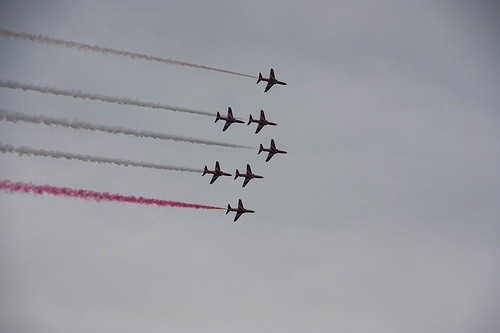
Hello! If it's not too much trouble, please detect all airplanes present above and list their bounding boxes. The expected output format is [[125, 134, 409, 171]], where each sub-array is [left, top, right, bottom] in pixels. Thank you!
[[201, 68, 288, 223]]
